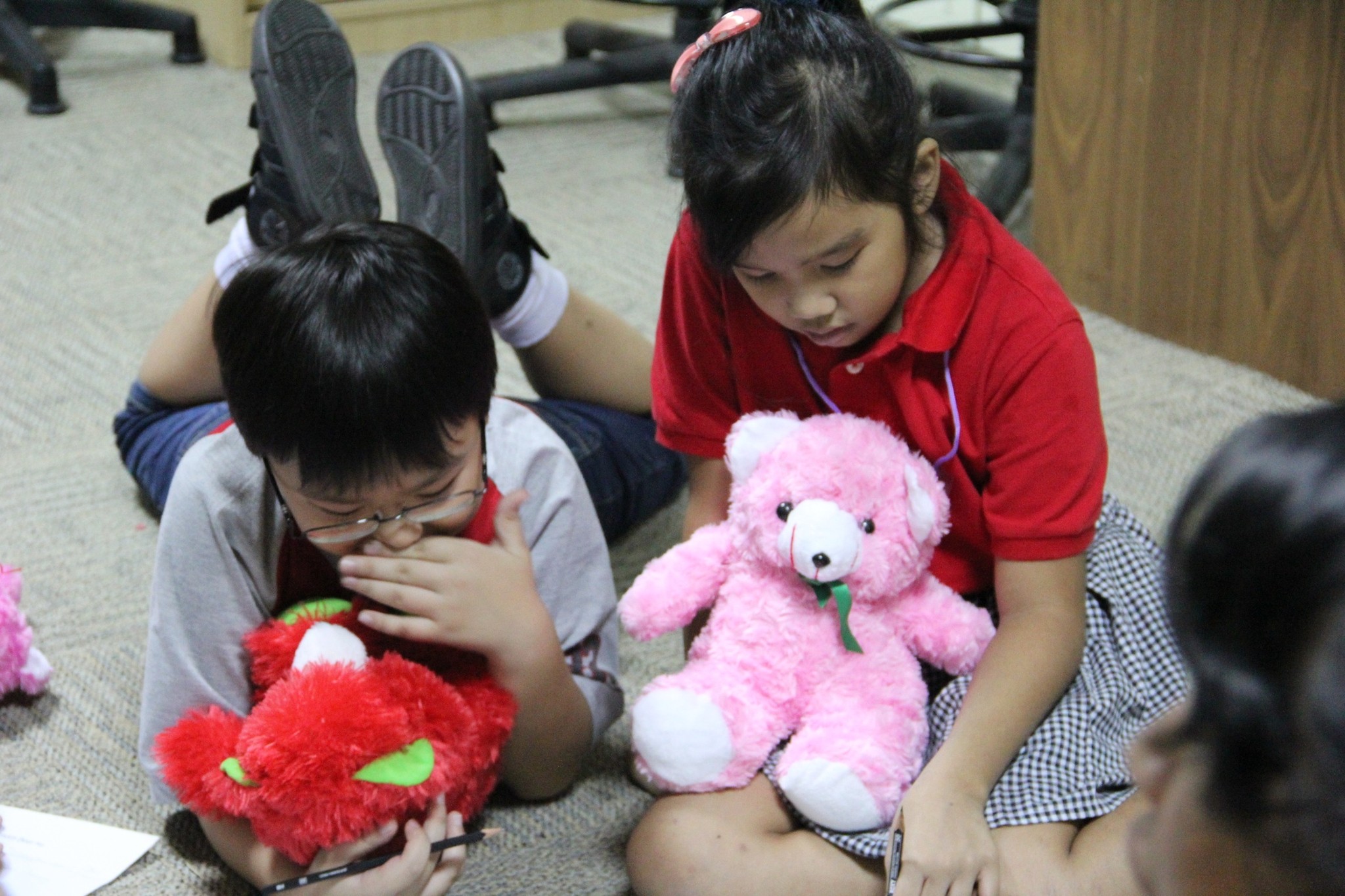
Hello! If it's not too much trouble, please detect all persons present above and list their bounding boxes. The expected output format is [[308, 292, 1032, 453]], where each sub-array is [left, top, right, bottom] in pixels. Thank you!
[[114, 3, 707, 896], [1123, 396, 1344, 896], [620, 0, 1203, 896]]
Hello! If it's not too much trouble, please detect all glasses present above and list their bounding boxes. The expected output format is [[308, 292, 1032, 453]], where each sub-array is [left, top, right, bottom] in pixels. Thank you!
[[259, 409, 488, 543]]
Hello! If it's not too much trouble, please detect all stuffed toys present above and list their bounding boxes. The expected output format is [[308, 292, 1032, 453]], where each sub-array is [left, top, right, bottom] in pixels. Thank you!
[[0, 564, 54, 704], [613, 403, 997, 835], [152, 587, 519, 873]]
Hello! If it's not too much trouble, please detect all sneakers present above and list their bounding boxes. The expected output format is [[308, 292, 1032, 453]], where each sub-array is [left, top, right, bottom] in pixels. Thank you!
[[376, 41, 550, 322], [206, 1, 381, 250]]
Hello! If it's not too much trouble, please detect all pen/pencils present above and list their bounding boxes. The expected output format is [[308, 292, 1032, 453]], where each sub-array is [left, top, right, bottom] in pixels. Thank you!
[[255, 827, 504, 896]]
